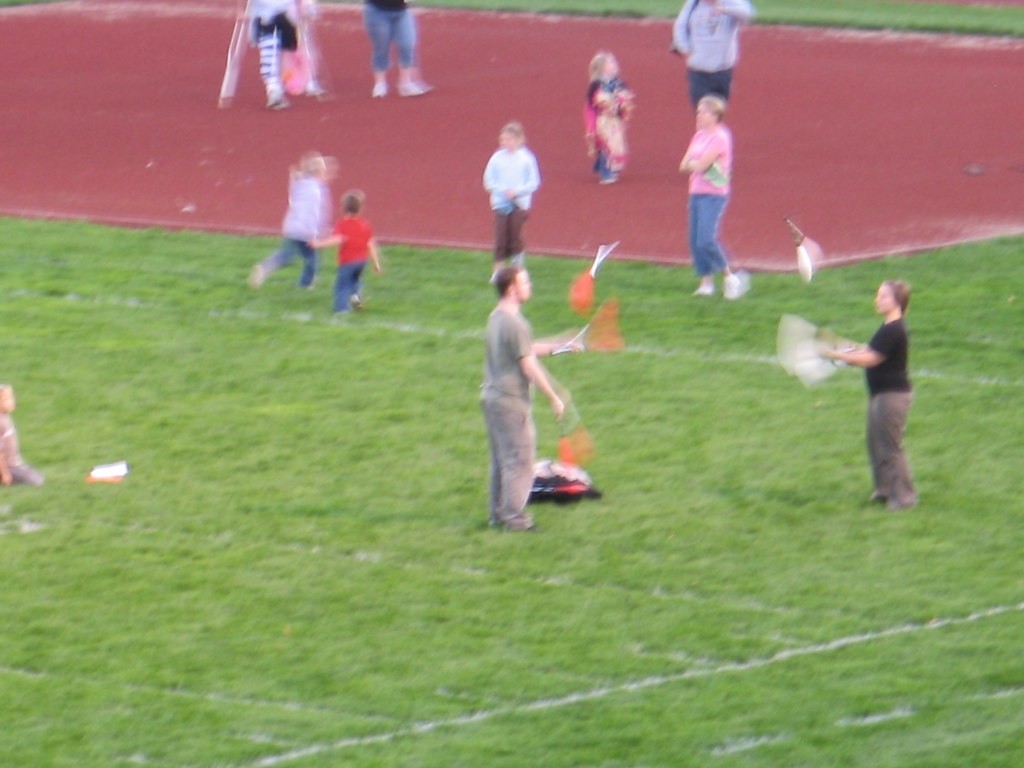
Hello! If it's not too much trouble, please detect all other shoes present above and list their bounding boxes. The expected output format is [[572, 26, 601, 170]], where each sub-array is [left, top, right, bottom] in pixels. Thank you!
[[692, 284, 714, 298], [723, 275, 742, 301], [400, 83, 424, 97], [372, 81, 386, 97]]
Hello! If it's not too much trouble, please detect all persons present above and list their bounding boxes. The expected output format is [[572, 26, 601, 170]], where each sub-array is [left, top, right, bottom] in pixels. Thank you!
[[360, 0, 428, 97], [821, 281, 917, 512], [480, 267, 586, 533], [679, 95, 744, 299], [249, 151, 381, 320], [483, 122, 540, 283], [245, 0, 326, 109], [672, 0, 754, 107], [584, 51, 634, 185], [0, 385, 43, 487]]
